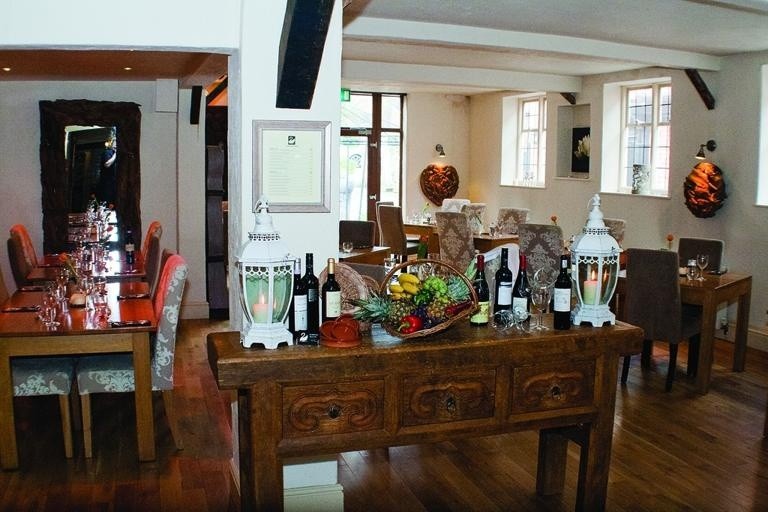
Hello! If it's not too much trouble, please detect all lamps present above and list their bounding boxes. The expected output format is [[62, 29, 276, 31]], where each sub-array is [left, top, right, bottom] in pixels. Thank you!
[[434, 143, 447, 158], [694, 139, 717, 161]]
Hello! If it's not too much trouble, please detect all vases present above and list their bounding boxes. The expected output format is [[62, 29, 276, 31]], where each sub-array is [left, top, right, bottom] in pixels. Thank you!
[[74, 275, 87, 295]]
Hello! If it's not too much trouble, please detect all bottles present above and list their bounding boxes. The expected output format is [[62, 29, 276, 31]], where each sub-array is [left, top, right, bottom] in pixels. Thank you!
[[493, 247, 512, 314], [302, 253, 319, 335], [469, 255, 490, 328], [554, 255, 573, 331], [511, 254, 532, 323], [321, 258, 341, 325], [124, 231, 135, 264], [289, 257, 309, 341]]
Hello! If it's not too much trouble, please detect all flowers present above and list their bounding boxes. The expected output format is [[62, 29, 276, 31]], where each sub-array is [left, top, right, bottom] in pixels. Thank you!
[[550, 215, 559, 226], [103, 227, 114, 233], [104, 204, 115, 213], [55, 252, 80, 278], [667, 233, 675, 251], [91, 193, 99, 207]]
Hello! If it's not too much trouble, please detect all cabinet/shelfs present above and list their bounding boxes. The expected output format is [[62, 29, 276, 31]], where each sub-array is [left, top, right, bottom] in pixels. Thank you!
[[66, 127, 115, 206]]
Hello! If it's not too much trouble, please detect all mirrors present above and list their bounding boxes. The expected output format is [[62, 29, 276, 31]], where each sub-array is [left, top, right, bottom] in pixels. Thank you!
[[36, 99, 143, 252]]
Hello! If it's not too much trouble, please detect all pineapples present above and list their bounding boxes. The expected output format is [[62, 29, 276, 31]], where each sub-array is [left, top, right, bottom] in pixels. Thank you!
[[425, 258, 477, 324], [341, 287, 417, 328]]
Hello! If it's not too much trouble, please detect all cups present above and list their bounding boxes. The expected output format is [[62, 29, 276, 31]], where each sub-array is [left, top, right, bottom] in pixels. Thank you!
[[687, 265, 699, 281], [487, 215, 503, 238], [384, 258, 396, 277], [93, 295, 108, 307], [82, 262, 92, 283], [513, 307, 538, 334], [53, 288, 63, 305], [490, 307, 523, 333], [424, 211, 432, 223], [81, 250, 93, 267], [343, 242, 353, 253], [44, 281, 59, 303], [56, 271, 68, 295], [40, 296, 60, 327], [95, 275, 106, 297], [697, 254, 709, 281], [531, 287, 550, 332], [412, 212, 418, 223], [78, 278, 93, 313]]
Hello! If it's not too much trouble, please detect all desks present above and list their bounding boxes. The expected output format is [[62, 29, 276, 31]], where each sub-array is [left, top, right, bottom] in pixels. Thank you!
[[206, 322, 645, 511], [68, 211, 117, 225], [67, 226, 119, 244], [0, 282, 157, 471], [26, 253, 145, 280], [611, 267, 754, 395]]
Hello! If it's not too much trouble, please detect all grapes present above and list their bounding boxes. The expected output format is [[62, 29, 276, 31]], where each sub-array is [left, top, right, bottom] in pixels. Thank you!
[[409, 301, 445, 331]]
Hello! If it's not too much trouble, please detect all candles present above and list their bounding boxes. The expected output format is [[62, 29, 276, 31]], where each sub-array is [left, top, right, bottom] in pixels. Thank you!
[[252, 304, 271, 321]]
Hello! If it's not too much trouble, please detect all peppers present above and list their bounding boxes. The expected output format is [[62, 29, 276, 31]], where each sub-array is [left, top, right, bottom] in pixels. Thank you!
[[397, 316, 421, 334]]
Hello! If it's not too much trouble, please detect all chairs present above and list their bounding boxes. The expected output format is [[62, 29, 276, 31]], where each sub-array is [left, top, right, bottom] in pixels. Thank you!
[[143, 221, 162, 261], [621, 248, 700, 392], [340, 221, 376, 248], [434, 211, 480, 275], [518, 224, 561, 279], [150, 248, 177, 304], [490, 207, 530, 234], [377, 205, 428, 274], [0, 268, 9, 309], [375, 200, 420, 264], [142, 233, 161, 289], [441, 199, 471, 213], [10, 235, 33, 283], [339, 261, 387, 294], [581, 218, 626, 246], [458, 203, 484, 234], [10, 223, 38, 268], [75, 255, 188, 457], [677, 237, 723, 288], [0, 355, 76, 460]]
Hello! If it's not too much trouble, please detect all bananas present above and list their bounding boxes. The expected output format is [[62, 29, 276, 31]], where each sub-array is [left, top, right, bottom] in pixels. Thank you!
[[388, 272, 421, 301]]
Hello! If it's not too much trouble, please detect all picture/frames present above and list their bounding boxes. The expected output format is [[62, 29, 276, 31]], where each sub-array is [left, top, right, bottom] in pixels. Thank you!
[[251, 119, 332, 214], [572, 127, 590, 173]]
